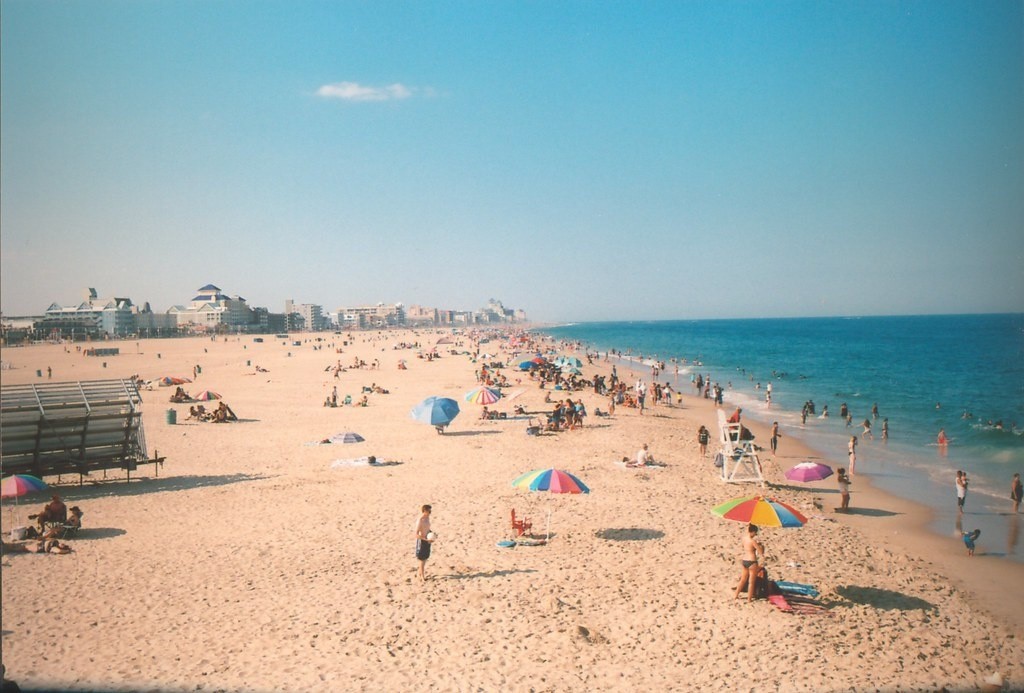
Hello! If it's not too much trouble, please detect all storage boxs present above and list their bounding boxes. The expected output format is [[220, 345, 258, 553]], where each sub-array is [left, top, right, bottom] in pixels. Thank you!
[[528, 426, 539, 434]]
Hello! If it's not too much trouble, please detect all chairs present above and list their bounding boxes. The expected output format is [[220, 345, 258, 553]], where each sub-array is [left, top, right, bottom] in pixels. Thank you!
[[718, 409, 755, 444], [511, 508, 532, 535], [38, 505, 83, 538]]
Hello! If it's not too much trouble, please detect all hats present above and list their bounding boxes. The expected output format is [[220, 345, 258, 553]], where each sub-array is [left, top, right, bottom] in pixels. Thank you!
[[70, 506, 78, 511]]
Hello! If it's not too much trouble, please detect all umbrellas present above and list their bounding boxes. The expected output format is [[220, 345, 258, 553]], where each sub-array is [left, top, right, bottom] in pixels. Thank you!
[[710, 494, 808, 561], [0, 474, 50, 526], [329, 432, 365, 444], [408, 396, 460, 426], [464, 386, 500, 409], [191, 390, 222, 401], [784, 461, 834, 509], [512, 469, 591, 542]]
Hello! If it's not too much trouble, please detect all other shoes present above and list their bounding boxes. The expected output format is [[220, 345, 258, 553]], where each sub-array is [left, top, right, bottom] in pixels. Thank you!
[[28, 514, 37, 519]]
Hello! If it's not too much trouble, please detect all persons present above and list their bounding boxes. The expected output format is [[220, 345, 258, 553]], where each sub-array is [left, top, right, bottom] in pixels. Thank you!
[[962, 529, 981, 556], [1, 327, 1024, 512], [731, 522, 775, 603], [416, 504, 438, 582], [38, 494, 80, 551]]
